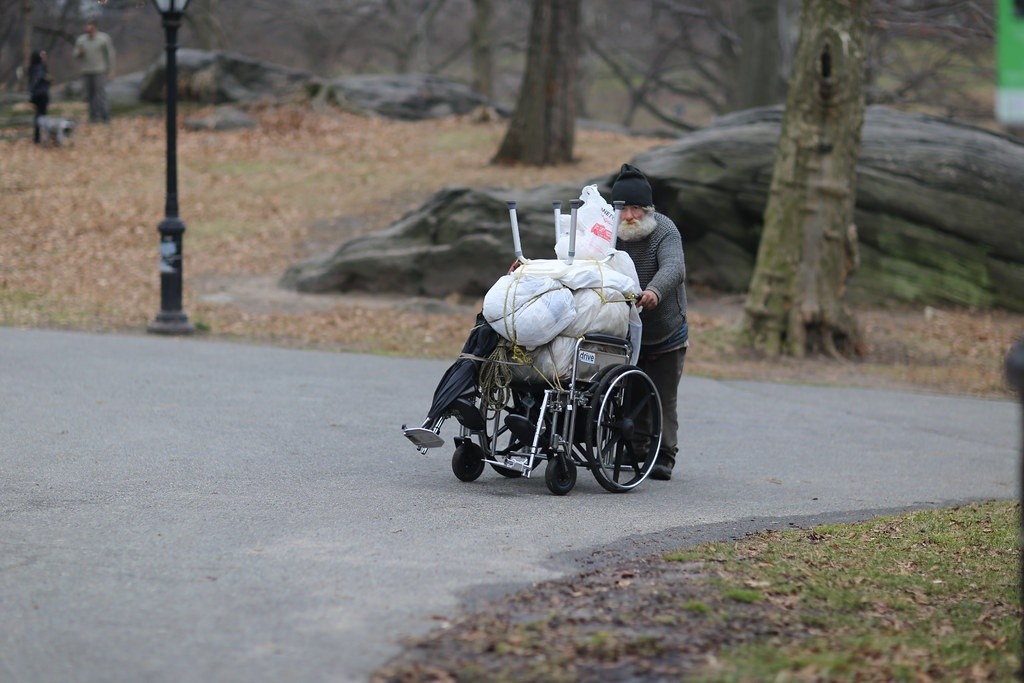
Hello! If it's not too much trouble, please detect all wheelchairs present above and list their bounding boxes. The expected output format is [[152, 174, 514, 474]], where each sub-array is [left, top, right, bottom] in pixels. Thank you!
[[400, 294, 663, 495]]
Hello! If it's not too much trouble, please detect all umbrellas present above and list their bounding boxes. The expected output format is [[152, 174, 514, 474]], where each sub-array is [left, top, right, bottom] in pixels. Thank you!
[[418, 256, 525, 429]]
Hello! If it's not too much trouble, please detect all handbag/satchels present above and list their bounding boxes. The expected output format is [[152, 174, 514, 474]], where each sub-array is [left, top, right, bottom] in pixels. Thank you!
[[554, 184, 616, 260]]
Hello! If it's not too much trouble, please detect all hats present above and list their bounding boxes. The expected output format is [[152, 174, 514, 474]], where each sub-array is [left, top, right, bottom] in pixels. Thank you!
[[611, 163, 653, 207]]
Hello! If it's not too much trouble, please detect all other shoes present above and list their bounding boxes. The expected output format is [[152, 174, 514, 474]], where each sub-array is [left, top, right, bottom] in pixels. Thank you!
[[612, 447, 649, 465], [647, 459, 673, 480]]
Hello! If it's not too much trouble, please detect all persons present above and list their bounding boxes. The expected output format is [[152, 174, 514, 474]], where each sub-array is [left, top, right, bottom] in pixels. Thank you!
[[72, 15, 118, 126], [605, 162, 692, 480], [24, 47, 55, 150]]
